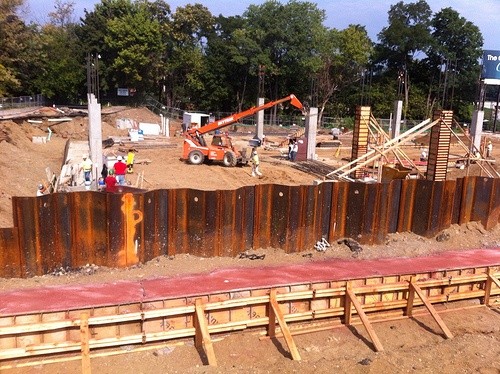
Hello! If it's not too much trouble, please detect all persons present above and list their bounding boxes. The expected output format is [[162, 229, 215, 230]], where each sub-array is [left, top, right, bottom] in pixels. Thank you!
[[114, 155, 127, 184], [286, 138, 298, 162], [487, 141, 492, 157], [422, 149, 429, 157], [250, 151, 262, 178], [126, 148, 135, 173], [331, 127, 341, 140], [105, 169, 117, 193], [37, 183, 48, 196], [101, 164, 108, 180], [80, 155, 93, 181]]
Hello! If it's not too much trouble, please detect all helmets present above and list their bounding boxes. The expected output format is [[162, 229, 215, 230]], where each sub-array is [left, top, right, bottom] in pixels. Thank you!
[[38, 184, 43, 189], [254, 151, 257, 154], [117, 156, 122, 160], [109, 170, 115, 174]]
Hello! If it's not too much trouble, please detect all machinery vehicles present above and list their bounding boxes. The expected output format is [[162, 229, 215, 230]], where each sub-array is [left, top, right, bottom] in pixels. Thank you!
[[182, 94, 303, 167]]
